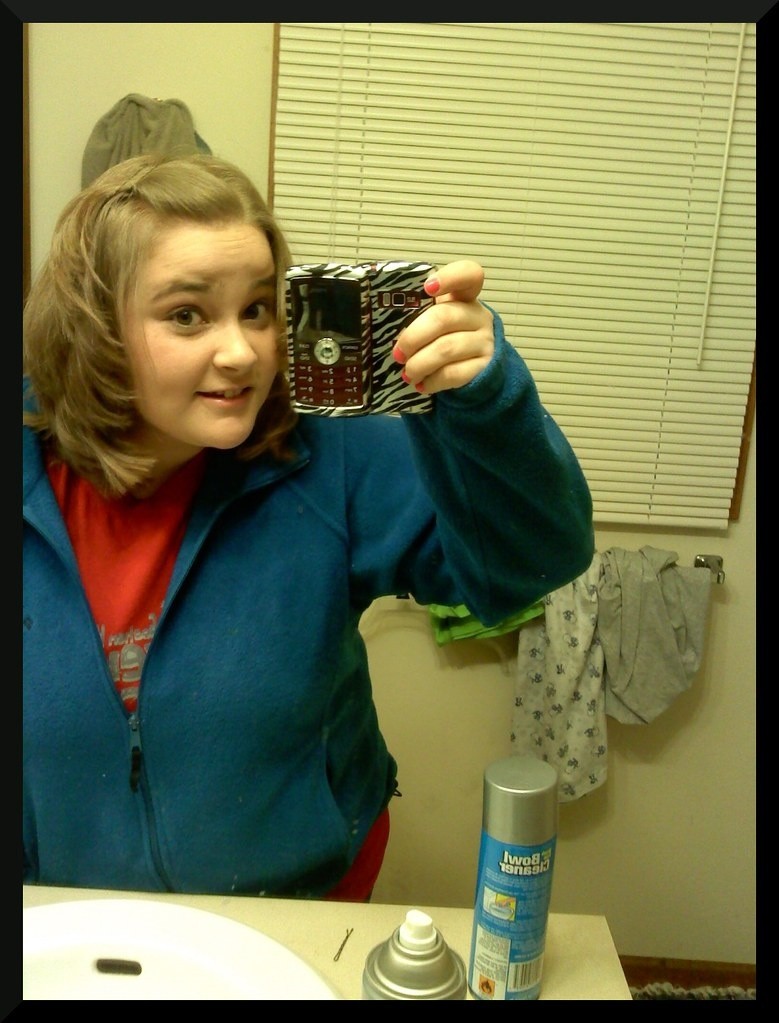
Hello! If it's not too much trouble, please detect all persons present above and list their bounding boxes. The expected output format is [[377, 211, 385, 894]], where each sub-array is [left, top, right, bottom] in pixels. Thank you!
[[25, 153, 594, 905]]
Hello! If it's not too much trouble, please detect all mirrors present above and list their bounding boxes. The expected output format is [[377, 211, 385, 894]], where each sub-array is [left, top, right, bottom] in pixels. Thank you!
[[25, 21, 755, 999]]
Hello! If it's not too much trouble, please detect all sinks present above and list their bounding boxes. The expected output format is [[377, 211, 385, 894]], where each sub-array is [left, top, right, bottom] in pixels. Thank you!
[[23, 899, 338, 1001]]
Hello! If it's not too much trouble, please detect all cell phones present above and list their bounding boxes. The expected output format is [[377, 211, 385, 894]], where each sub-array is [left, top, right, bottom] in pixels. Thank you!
[[285, 260, 437, 418]]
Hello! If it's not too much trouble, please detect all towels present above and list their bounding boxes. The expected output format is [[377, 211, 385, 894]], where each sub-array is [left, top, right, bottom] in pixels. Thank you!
[[509, 553, 608, 803], [81, 94, 197, 190]]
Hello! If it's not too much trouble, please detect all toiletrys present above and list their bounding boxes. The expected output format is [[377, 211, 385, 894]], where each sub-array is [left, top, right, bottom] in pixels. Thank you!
[[363, 909, 466, 1000], [468, 755, 558, 1000]]
